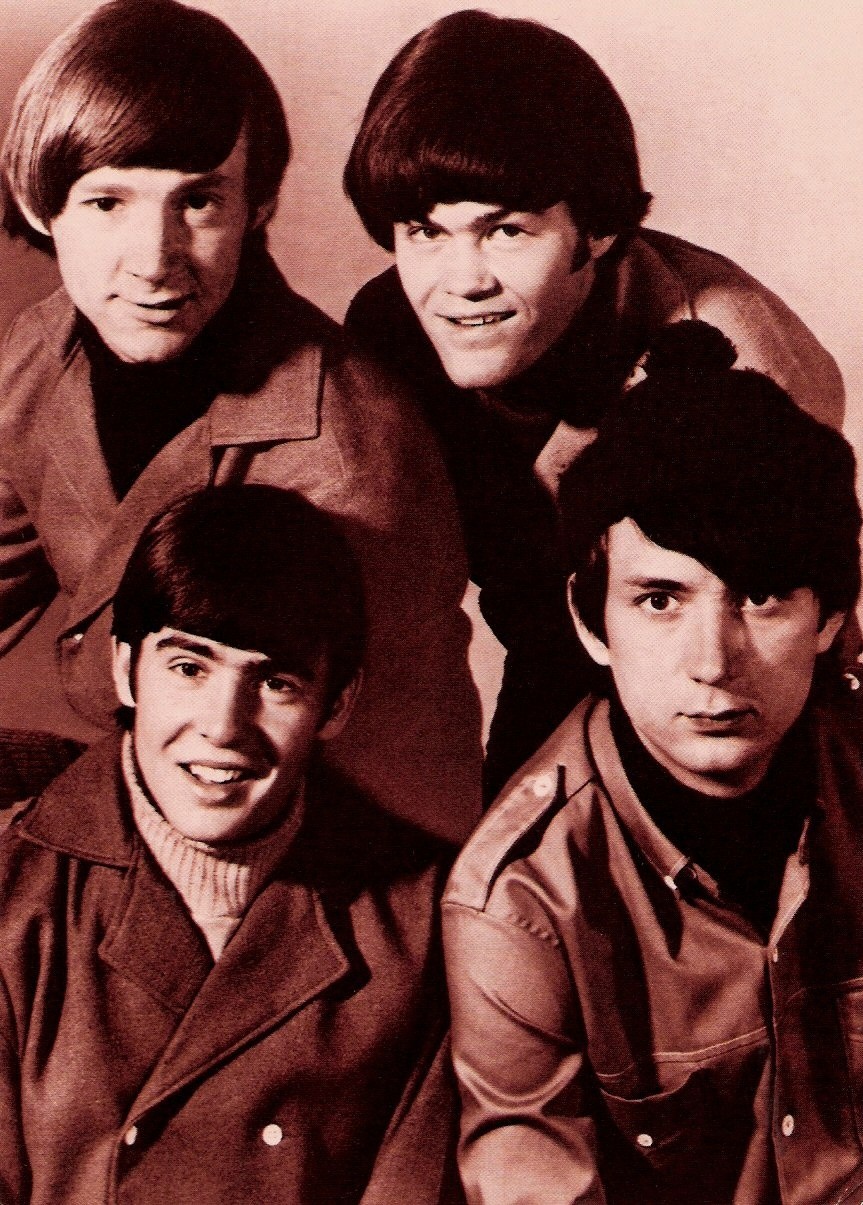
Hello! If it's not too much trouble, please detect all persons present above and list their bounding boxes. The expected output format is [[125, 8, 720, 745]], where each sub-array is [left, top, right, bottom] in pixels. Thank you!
[[444, 320, 863, 1205], [0, 0, 483, 849], [343, 10, 847, 818], [0, 481, 472, 1205]]
